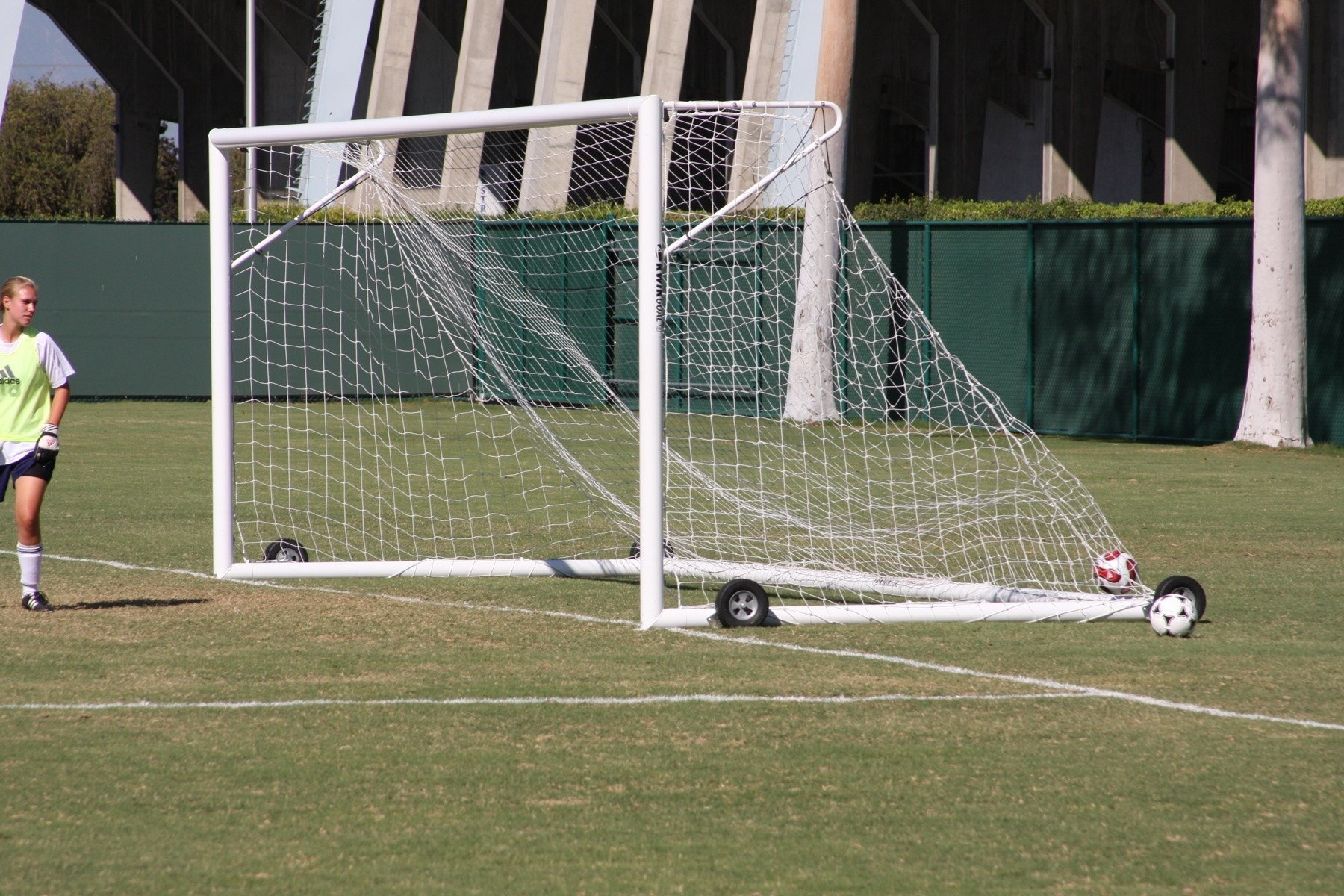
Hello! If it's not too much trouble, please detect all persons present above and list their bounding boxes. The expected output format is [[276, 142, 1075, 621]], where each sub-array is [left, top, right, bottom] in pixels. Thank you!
[[0, 276, 76, 613]]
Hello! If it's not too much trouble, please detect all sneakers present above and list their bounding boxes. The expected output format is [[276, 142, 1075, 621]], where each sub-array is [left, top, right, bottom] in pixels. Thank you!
[[22, 591, 54, 613]]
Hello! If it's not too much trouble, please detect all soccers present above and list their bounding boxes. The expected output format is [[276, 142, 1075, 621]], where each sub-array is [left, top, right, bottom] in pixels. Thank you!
[[1148, 593, 1198, 638], [1094, 550, 1136, 594]]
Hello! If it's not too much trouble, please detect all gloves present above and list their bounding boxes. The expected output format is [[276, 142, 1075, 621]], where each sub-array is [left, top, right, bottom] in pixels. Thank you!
[[34, 423, 59, 468]]
